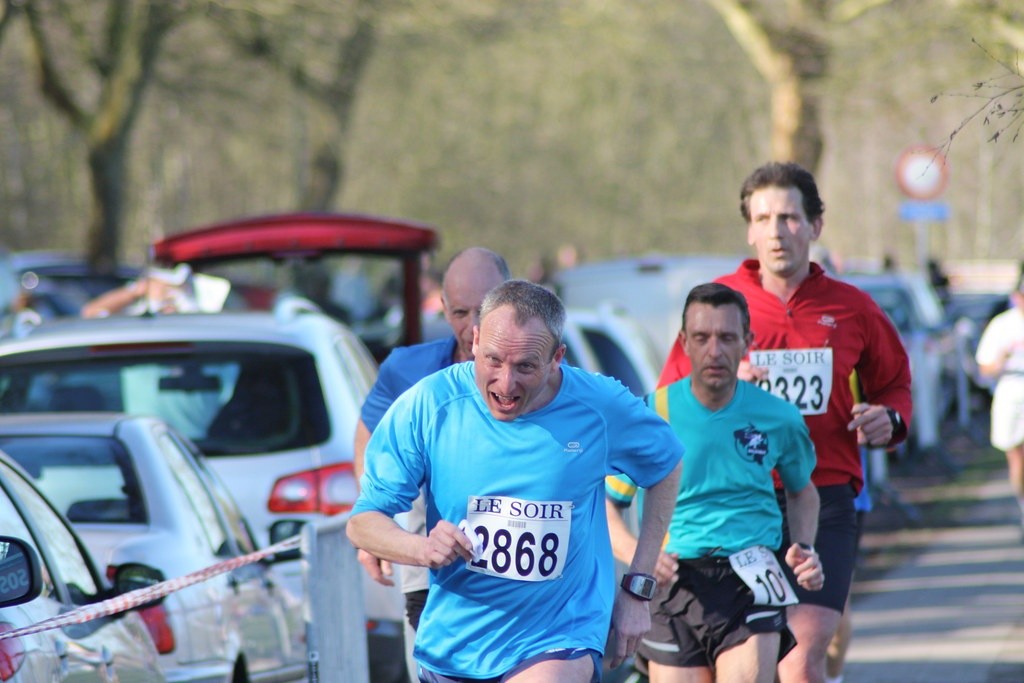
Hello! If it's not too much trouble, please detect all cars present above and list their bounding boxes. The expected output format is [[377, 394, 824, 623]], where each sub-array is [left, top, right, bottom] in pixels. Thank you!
[[557, 252, 1012, 458], [0, 214, 453, 683]]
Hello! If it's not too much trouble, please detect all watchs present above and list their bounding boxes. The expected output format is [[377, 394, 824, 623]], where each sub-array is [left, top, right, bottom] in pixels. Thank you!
[[621, 569, 658, 603], [886, 410, 902, 439]]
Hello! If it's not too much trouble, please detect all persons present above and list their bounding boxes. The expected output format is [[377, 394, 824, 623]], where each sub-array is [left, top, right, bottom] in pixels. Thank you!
[[652, 160, 912, 683], [974, 261, 1024, 541], [12, 245, 684, 683], [605, 281, 827, 683]]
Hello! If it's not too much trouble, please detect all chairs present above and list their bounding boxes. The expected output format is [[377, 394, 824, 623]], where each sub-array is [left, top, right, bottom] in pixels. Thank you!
[[66, 501, 134, 522], [202, 361, 292, 439]]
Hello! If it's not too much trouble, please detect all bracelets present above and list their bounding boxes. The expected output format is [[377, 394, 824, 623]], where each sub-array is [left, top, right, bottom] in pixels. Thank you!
[[799, 542, 816, 553]]
